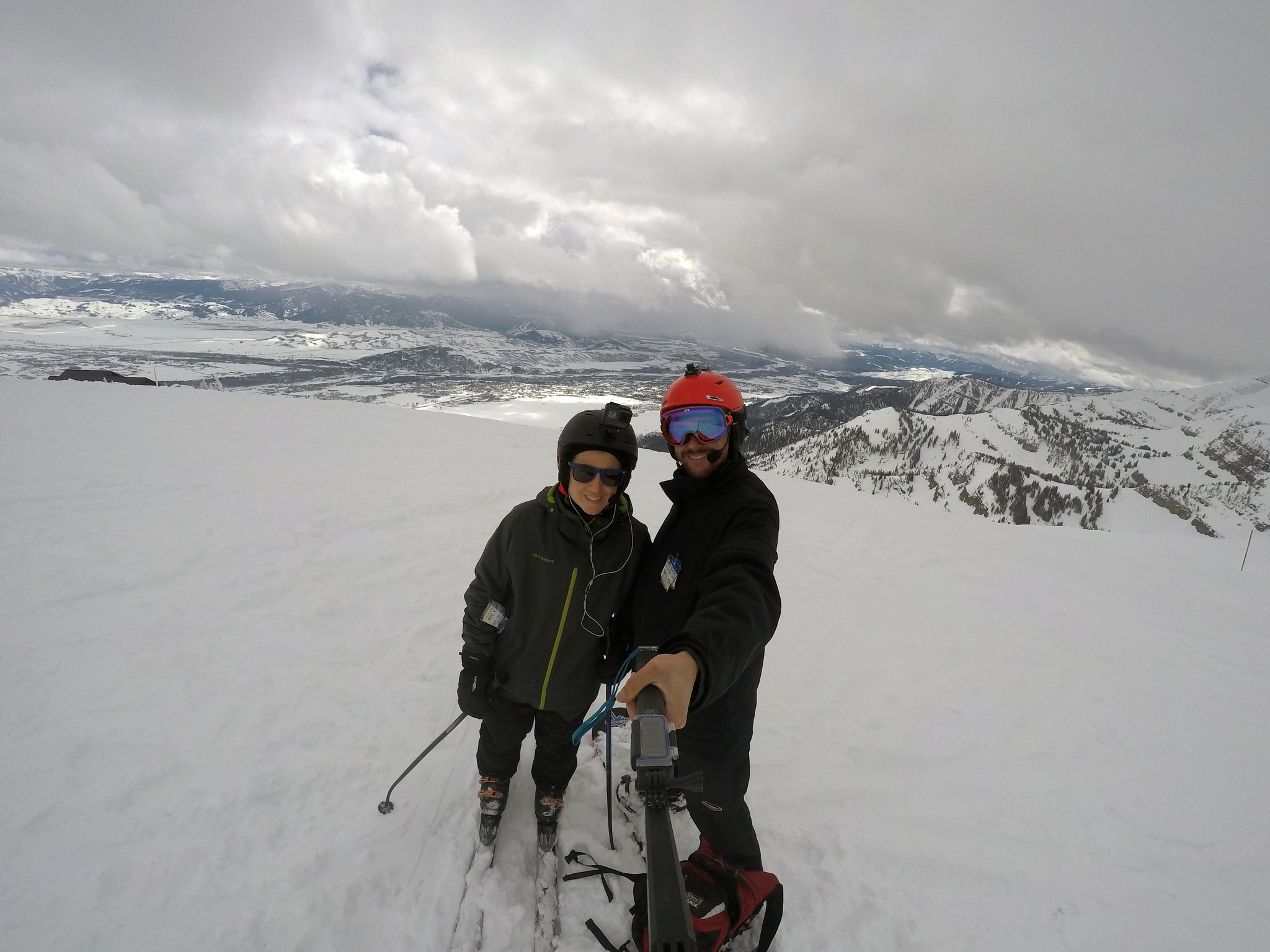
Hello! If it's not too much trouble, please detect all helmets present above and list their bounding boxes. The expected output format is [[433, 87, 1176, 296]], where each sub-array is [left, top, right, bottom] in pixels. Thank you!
[[660, 371, 747, 460], [557, 410, 638, 488]]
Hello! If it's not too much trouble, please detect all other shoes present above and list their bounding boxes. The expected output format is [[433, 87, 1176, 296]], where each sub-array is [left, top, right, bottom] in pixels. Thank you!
[[479, 775, 509, 816], [668, 791, 687, 814], [533, 786, 562, 823]]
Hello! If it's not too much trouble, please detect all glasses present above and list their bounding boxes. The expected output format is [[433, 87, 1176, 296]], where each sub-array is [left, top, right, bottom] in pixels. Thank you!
[[568, 461, 627, 487], [660, 406, 728, 446]]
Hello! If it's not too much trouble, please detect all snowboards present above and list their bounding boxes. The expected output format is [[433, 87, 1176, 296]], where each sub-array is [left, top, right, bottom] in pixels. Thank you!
[[592, 707, 646, 863]]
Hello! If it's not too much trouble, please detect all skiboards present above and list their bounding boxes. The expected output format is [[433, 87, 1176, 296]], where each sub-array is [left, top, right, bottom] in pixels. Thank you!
[[449, 812, 561, 952]]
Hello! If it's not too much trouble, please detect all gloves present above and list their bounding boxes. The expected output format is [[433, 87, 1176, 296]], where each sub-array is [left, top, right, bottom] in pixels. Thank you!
[[457, 652, 489, 720], [597, 630, 634, 686]]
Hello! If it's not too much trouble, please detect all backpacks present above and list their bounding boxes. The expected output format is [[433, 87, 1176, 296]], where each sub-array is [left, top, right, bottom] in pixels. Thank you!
[[630, 834, 783, 952]]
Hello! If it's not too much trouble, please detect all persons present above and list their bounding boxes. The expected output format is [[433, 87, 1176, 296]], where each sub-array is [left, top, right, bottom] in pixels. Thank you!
[[457, 401, 655, 854], [615, 363, 780, 915]]
